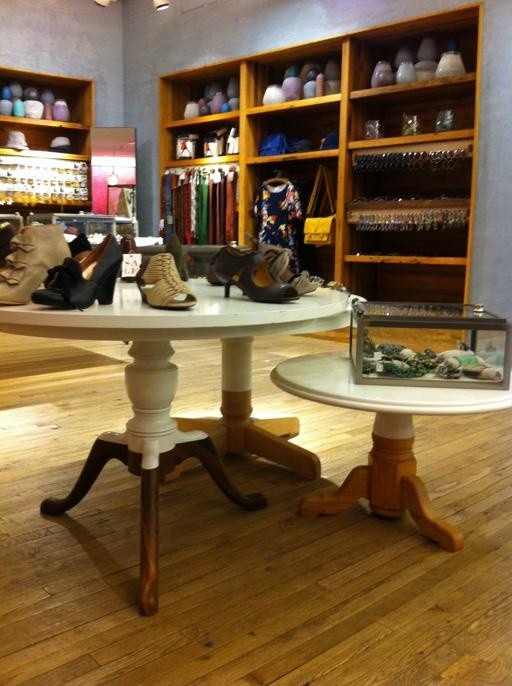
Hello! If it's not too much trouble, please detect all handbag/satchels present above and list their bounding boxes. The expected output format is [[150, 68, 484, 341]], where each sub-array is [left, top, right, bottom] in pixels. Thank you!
[[202, 127, 227, 157], [258, 133, 289, 156], [174, 132, 199, 160], [433, 108, 456, 133], [320, 130, 339, 151], [398, 113, 421, 136], [287, 136, 314, 154], [302, 212, 339, 250], [225, 127, 241, 155], [361, 117, 384, 140]]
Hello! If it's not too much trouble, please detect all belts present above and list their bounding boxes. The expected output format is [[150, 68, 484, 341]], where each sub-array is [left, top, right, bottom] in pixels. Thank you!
[[158, 164, 239, 247]]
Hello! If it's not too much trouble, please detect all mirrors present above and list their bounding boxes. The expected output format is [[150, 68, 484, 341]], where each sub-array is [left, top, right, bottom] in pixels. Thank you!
[[92, 127, 138, 217]]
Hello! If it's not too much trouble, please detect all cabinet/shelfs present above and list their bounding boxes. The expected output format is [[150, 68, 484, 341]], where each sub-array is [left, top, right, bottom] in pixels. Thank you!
[[158, 0, 485, 352], [1, 66, 97, 215]]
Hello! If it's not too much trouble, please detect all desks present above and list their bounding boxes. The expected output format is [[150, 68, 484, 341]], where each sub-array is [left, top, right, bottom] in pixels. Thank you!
[[0, 278, 360, 617], [185, 278, 366, 481], [271, 351, 512, 551]]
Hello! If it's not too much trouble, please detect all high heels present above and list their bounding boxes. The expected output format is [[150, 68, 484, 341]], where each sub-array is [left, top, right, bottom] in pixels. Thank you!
[[207, 242, 324, 303], [0, 219, 92, 311], [164, 232, 189, 281], [30, 232, 123, 309], [120, 232, 139, 282]]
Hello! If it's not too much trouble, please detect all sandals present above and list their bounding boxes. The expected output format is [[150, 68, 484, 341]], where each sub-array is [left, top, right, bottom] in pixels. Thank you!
[[135, 251, 198, 310]]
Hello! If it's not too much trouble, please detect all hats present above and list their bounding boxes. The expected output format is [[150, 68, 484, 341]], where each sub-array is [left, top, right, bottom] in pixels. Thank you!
[[6, 129, 31, 151], [49, 136, 73, 154]]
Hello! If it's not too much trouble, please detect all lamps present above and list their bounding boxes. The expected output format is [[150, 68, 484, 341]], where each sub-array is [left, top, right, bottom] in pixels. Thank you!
[[153, 0, 170, 11], [93, 0, 109, 7], [106, 147, 120, 187]]
